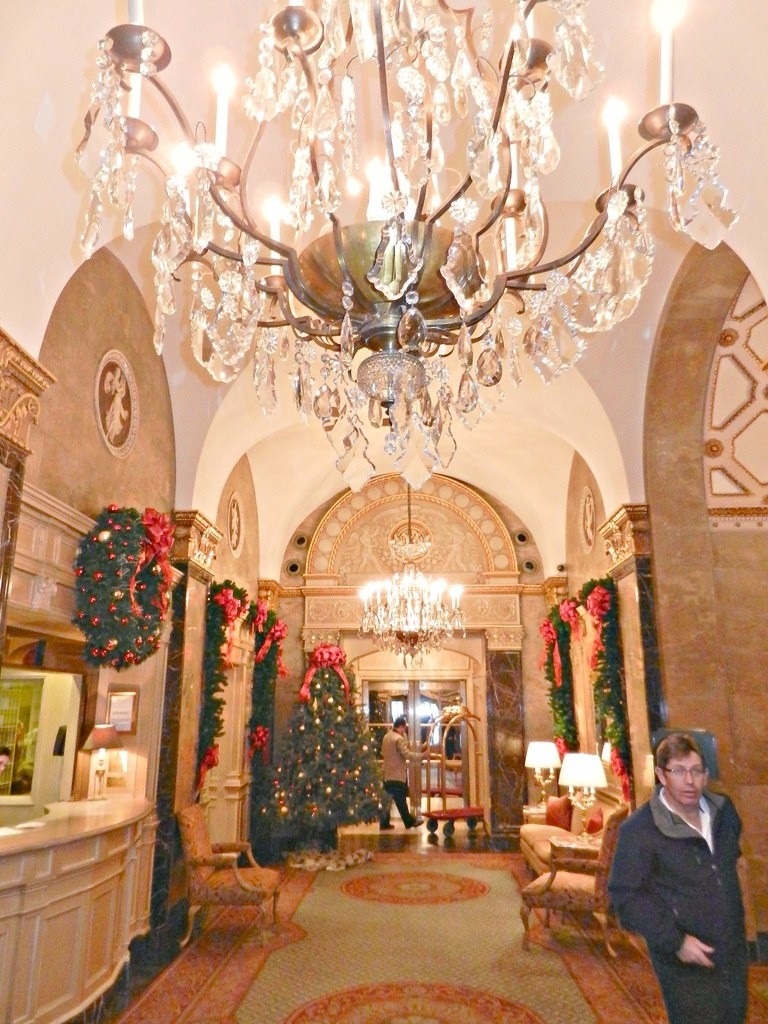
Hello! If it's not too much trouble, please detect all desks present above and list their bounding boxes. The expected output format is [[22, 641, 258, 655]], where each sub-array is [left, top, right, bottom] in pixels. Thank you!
[[521, 803, 548, 825], [550, 835, 601, 877]]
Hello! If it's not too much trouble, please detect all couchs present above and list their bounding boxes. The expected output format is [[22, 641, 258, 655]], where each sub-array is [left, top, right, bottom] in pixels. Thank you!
[[519, 786, 624, 876]]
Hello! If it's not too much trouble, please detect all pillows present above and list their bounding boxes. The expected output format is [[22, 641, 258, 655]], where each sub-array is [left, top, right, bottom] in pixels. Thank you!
[[545, 796, 571, 830], [585, 807, 603, 833]]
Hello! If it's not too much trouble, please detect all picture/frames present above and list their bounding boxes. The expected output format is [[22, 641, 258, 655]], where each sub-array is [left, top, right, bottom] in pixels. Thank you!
[[105, 689, 138, 736]]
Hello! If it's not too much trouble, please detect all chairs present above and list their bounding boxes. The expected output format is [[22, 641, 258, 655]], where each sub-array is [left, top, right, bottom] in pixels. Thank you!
[[519, 806, 629, 958], [177, 801, 280, 947], [435, 754, 464, 783]]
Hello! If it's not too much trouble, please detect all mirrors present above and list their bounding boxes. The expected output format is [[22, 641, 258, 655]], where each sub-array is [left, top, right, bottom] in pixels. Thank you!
[[0, 673, 50, 807], [585, 674, 628, 808]]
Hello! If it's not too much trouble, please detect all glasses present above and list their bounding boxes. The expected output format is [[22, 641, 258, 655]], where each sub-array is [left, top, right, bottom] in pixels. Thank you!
[[665, 764, 704, 778]]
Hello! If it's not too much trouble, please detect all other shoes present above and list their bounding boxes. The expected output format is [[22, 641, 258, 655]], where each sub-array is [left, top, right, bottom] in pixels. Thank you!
[[380, 825, 394, 831], [406, 820, 424, 829]]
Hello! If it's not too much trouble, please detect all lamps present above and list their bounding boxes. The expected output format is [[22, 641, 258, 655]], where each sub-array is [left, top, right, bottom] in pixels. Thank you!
[[356, 477, 468, 669], [75, 0, 738, 489], [80, 724, 122, 800], [558, 754, 608, 843], [524, 742, 562, 811], [600, 739, 615, 764]]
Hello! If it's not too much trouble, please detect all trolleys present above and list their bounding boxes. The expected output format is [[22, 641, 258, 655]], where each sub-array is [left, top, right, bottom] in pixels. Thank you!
[[421, 712, 485, 838]]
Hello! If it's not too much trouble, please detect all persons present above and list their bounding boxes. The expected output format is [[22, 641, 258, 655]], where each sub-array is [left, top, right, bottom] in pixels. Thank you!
[[0, 746, 11, 772], [606, 733, 750, 1024], [378, 717, 429, 830]]
